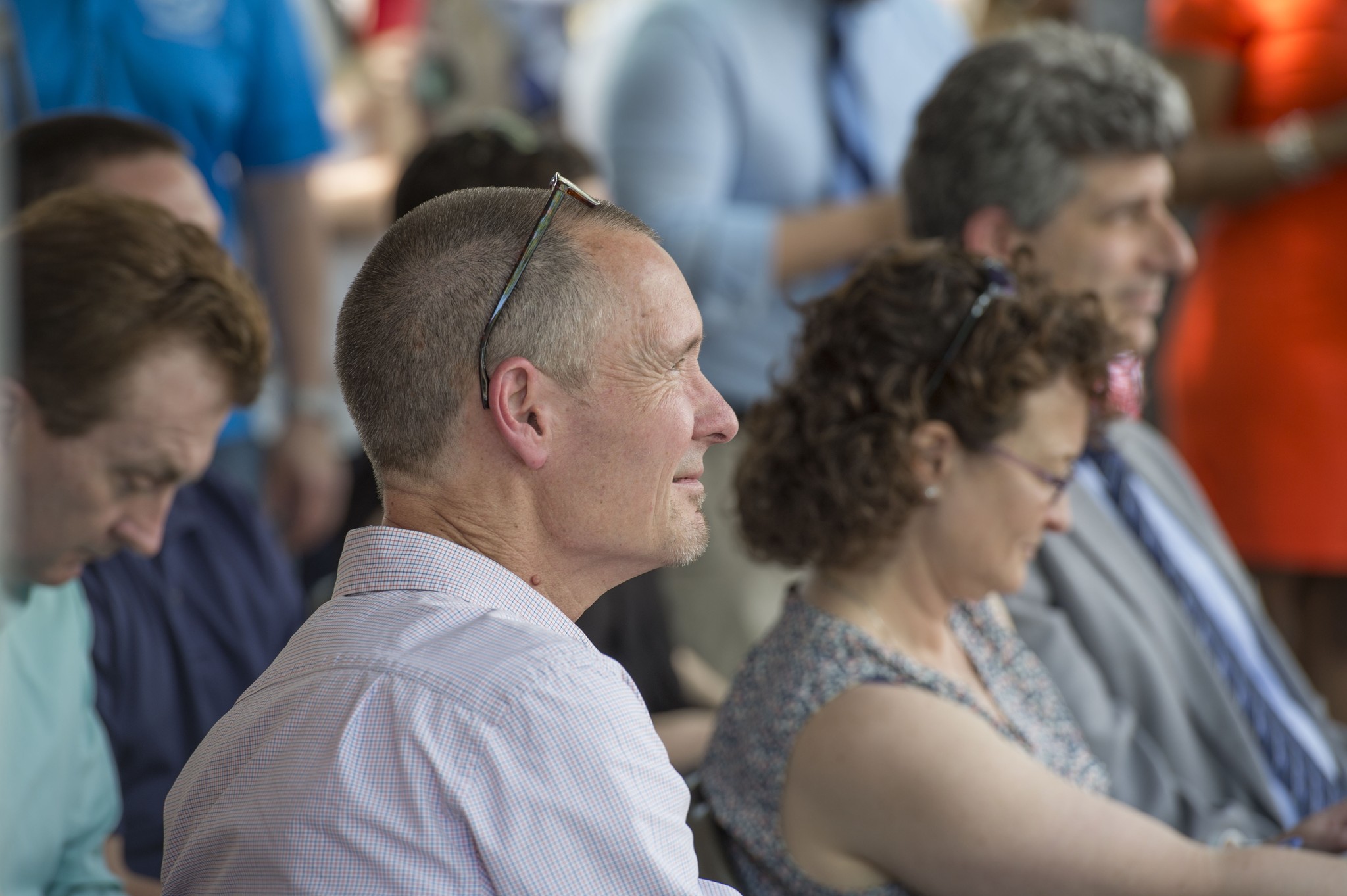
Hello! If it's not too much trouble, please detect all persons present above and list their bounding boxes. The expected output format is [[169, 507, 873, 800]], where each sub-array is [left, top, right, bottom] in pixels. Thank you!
[[160, 172, 743, 896], [295, 0, 1346, 896], [0, 1, 336, 895]]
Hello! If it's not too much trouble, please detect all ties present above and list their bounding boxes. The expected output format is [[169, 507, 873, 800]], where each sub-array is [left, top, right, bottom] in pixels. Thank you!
[[1086, 428, 1337, 821], [825, 0, 885, 199]]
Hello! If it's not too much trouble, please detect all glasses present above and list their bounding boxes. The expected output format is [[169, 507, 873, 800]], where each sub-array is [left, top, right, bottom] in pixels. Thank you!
[[479, 169, 599, 408], [929, 260, 1013, 403], [976, 447, 1077, 511]]
